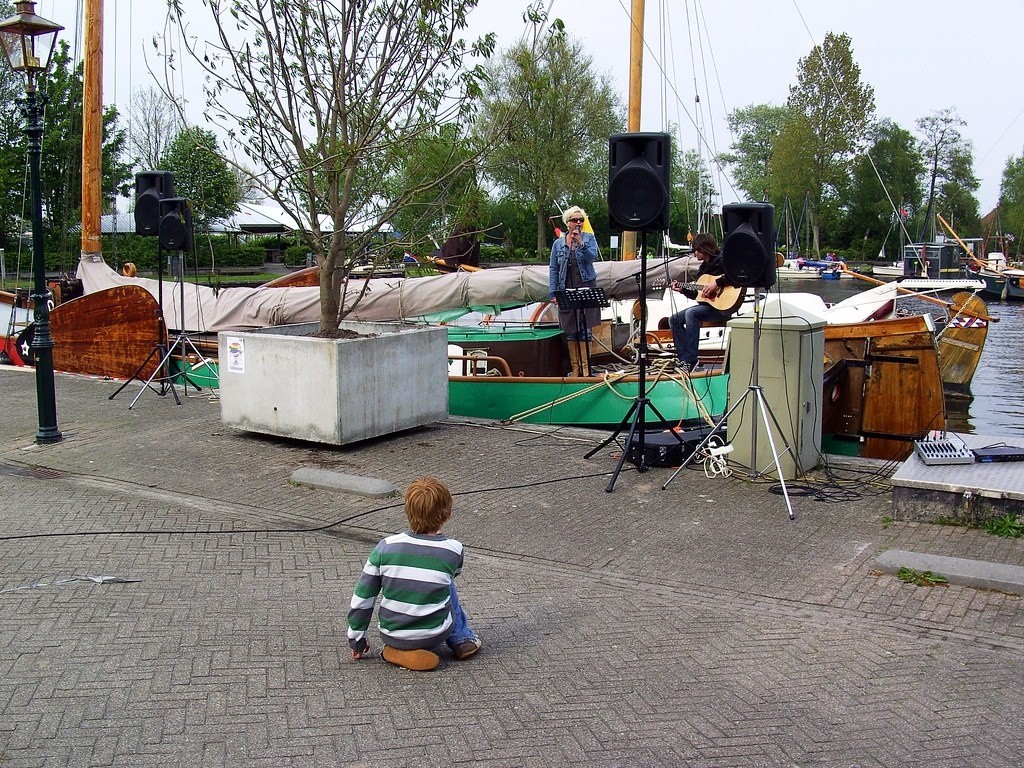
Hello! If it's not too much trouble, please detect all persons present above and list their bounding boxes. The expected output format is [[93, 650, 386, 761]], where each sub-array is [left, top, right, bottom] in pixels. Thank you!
[[821, 252, 847, 270], [548, 207, 602, 376], [347, 476, 481, 671], [668, 233, 746, 374]]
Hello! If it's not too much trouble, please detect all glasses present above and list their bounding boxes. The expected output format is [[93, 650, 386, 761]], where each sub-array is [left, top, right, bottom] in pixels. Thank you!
[[691, 248, 701, 252], [567, 217, 584, 223]]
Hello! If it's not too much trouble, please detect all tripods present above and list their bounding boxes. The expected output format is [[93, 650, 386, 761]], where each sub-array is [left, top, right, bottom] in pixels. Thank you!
[[111, 250, 219, 411], [583, 232, 805, 521]]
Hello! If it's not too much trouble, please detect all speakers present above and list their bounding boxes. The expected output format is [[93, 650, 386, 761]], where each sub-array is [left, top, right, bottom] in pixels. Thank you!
[[722, 201, 776, 290], [159, 197, 194, 251], [609, 132, 671, 233], [135, 171, 173, 239]]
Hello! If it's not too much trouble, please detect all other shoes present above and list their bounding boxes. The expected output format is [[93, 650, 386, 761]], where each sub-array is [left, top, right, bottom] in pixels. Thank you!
[[382, 645, 439, 670], [453, 635, 481, 659]]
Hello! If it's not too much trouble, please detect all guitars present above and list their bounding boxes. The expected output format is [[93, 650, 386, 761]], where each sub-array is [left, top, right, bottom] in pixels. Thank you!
[[650, 272, 747, 317]]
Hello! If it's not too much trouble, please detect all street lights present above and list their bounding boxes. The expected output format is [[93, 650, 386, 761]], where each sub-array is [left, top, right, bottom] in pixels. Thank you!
[[0, 0, 66, 447]]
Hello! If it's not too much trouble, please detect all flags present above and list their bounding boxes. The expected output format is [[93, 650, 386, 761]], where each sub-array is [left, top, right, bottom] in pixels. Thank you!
[[402, 253, 423, 267]]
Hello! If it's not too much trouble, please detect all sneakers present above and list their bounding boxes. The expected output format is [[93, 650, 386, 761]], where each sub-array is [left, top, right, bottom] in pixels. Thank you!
[[681, 359, 699, 374]]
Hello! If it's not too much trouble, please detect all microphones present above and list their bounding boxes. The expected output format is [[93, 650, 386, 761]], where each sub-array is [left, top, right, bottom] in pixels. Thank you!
[[674, 249, 695, 254], [571, 225, 581, 243]]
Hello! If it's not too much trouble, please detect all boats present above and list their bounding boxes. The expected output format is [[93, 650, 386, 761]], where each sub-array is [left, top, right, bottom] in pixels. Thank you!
[[0, 0, 1024, 463], [845, 356, 847, 358]]
